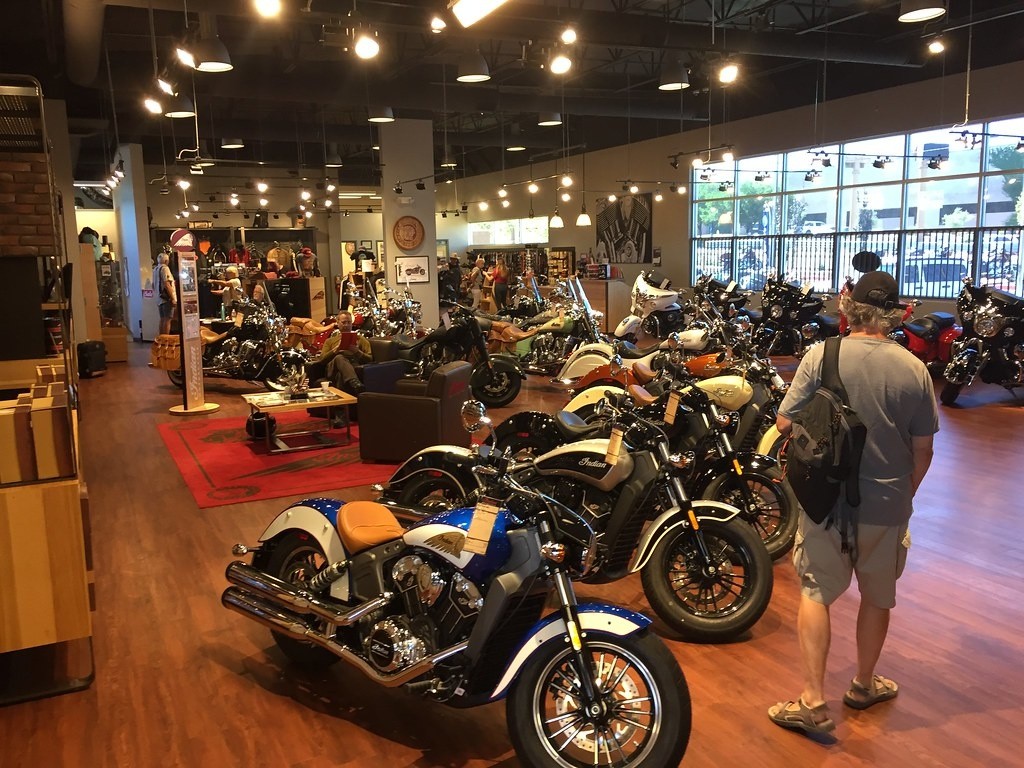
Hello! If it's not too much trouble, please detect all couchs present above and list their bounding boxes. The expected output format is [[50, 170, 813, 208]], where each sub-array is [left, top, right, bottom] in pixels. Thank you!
[[359, 359, 474, 460]]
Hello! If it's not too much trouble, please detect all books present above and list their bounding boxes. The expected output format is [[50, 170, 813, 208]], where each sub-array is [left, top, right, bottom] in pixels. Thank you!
[[340, 332, 360, 352]]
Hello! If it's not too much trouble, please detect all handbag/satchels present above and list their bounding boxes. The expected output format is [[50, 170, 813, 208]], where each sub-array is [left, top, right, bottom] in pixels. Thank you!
[[160, 287, 171, 301]]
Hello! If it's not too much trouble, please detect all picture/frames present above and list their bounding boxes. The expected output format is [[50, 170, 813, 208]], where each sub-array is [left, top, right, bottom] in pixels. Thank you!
[[395, 255, 430, 284]]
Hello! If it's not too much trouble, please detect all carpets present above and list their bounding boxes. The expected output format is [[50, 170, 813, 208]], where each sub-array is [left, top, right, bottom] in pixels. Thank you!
[[155, 410, 412, 508]]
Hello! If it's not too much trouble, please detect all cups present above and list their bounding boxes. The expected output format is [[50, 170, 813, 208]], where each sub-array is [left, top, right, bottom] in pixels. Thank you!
[[320, 381, 330, 392]]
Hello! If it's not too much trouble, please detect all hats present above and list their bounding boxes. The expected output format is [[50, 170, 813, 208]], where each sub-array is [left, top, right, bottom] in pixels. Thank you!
[[850, 271, 907, 310], [450, 253, 460, 258], [301, 247, 312, 257], [235, 241, 243, 251], [171, 229, 196, 253]]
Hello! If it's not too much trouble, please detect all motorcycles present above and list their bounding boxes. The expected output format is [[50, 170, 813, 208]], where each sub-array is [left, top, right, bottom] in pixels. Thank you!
[[716, 244, 805, 291], [835, 274, 964, 369], [368, 269, 860, 642], [333, 297, 526, 408], [148, 276, 310, 390], [939, 274, 1024, 403], [319, 274, 435, 340], [220, 399, 694, 768], [871, 245, 1024, 282]]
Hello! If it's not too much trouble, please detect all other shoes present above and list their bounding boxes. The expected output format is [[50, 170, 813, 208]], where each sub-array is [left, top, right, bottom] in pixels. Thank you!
[[333, 414, 346, 428], [348, 378, 367, 394]]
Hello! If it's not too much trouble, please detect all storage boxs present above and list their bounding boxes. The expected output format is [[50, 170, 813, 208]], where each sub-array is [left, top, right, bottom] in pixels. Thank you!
[[150, 334, 182, 371], [100, 326, 129, 362], [937, 326, 965, 361]]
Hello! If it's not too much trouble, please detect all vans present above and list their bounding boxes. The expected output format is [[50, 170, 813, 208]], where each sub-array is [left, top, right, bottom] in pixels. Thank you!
[[877, 259, 971, 299]]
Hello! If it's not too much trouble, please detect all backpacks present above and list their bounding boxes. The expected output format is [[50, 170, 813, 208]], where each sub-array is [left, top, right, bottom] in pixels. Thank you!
[[773, 336, 867, 554]]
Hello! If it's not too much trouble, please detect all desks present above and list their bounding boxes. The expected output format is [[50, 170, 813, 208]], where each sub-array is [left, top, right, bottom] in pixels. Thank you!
[[241, 386, 358, 453]]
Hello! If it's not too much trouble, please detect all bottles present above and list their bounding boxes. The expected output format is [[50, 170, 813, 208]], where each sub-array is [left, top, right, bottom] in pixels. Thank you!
[[221, 303, 236, 322]]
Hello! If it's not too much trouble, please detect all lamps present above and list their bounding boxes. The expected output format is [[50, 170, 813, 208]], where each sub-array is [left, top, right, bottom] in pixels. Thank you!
[[98, 0, 946, 230]]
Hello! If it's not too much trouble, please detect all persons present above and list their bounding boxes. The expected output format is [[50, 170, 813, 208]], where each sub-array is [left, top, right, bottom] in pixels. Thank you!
[[152, 253, 178, 336], [207, 265, 242, 318], [468, 257, 485, 311], [252, 284, 267, 306], [768, 270, 939, 734], [436, 253, 463, 302], [481, 259, 509, 310], [320, 311, 373, 428]]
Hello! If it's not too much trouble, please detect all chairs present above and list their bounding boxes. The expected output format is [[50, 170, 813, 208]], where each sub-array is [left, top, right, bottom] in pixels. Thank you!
[[305, 337, 404, 421]]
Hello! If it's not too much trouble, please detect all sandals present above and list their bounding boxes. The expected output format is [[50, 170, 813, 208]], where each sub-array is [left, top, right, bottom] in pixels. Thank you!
[[843, 673, 898, 709], [767, 697, 835, 734]]
